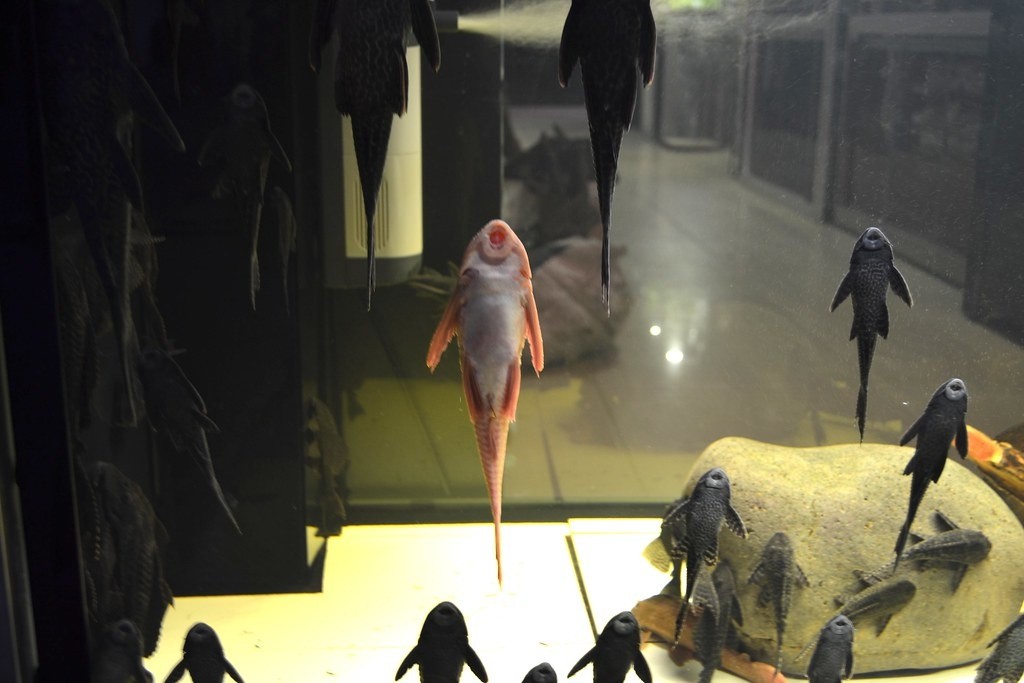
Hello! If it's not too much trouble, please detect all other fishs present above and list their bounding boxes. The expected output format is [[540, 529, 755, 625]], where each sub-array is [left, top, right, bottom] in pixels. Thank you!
[[0, 0, 1024, 683]]
